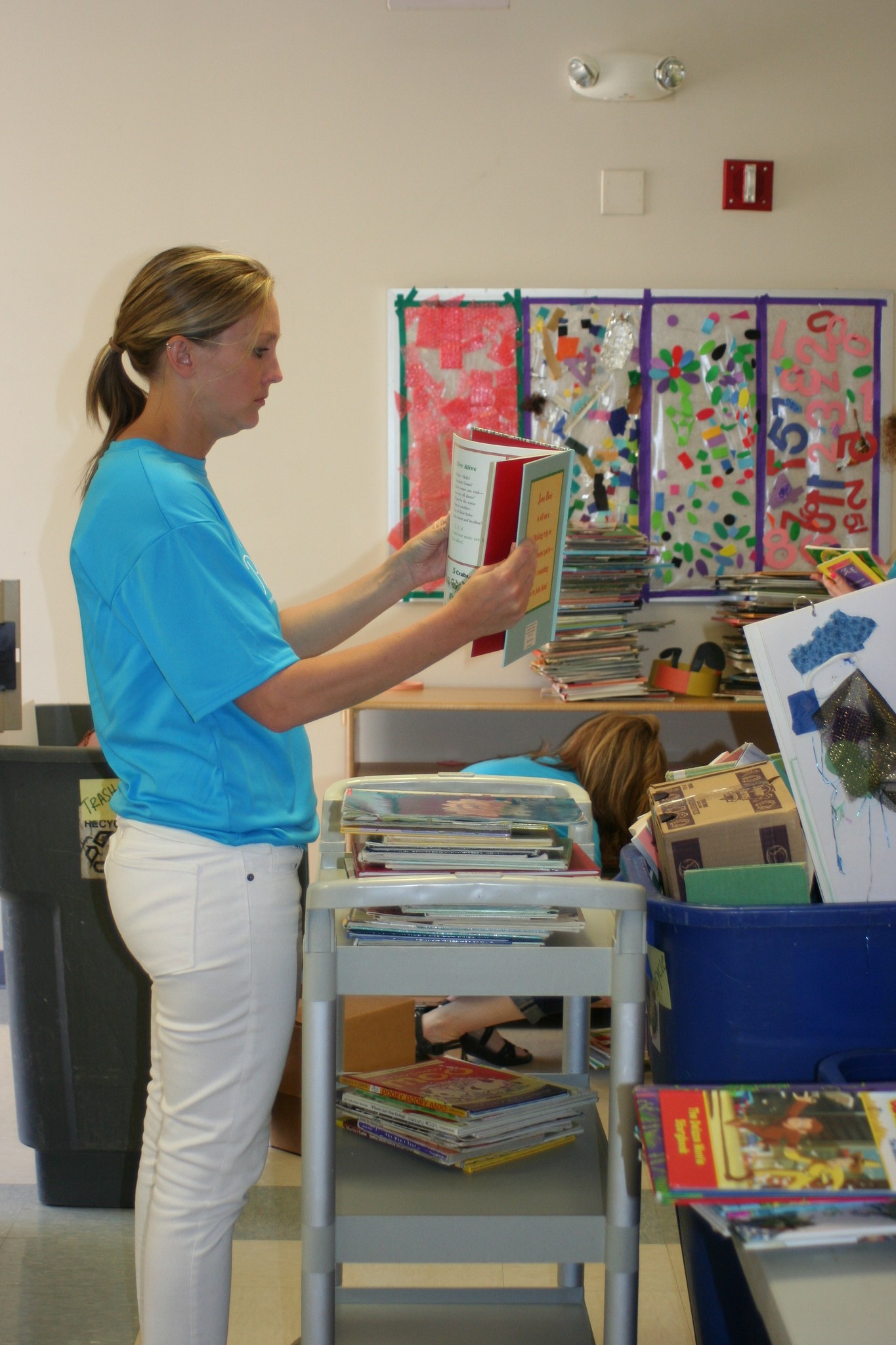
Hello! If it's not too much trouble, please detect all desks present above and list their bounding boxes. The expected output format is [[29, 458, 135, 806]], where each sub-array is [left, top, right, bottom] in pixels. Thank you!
[[346, 684, 778, 777]]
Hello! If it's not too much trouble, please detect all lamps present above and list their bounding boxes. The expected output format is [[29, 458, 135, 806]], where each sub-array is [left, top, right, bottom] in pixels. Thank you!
[[565, 52, 689, 103]]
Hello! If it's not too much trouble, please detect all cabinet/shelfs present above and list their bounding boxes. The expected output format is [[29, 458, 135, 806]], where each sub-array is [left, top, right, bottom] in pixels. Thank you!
[[291, 770, 654, 1345]]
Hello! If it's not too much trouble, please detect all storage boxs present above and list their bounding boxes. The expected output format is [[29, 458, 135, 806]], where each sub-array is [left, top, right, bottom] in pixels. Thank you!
[[647, 757, 808, 902]]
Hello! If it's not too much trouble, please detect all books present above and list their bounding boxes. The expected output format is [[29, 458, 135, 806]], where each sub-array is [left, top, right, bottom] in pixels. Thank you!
[[681, 858, 809, 907], [530, 522, 675, 703], [333, 786, 603, 948], [631, 740, 767, 887], [709, 543, 891, 701], [339, 1060, 603, 1173], [629, 1082, 894, 1255], [445, 427, 576, 668]]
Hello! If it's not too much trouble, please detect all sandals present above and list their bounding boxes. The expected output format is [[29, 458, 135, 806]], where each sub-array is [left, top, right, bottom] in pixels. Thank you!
[[414, 1009, 471, 1063], [433, 999, 532, 1065]]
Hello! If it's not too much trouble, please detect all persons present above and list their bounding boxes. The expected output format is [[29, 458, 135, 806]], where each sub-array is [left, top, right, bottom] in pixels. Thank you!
[[809, 415, 894, 598], [67, 244, 537, 1344], [414, 712, 672, 1068]]
